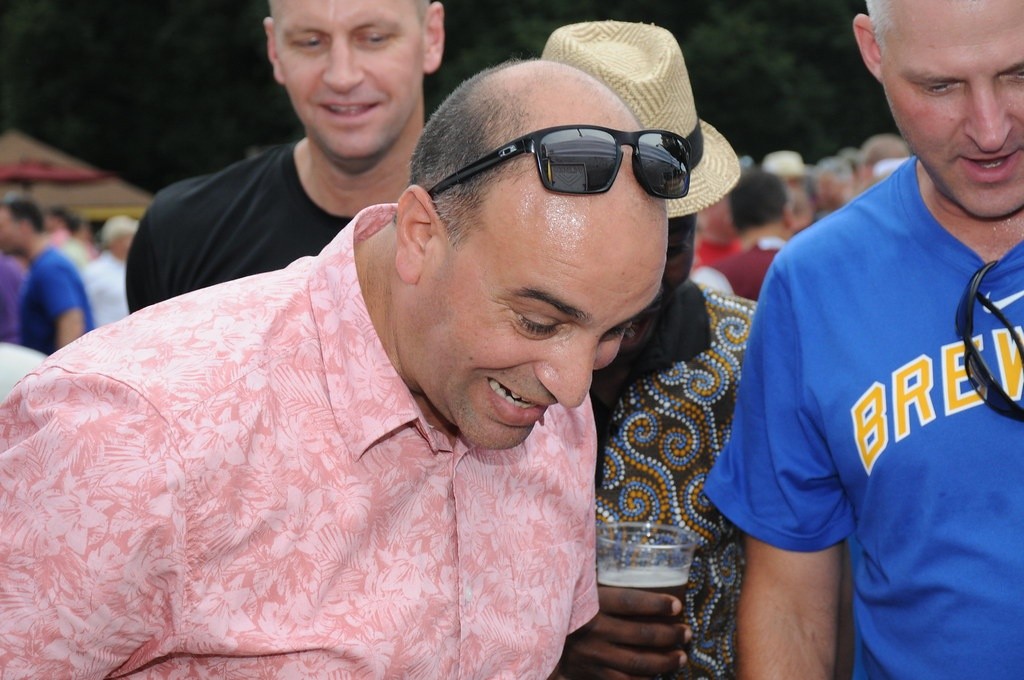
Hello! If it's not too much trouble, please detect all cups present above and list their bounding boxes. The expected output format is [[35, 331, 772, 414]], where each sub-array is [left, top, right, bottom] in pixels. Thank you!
[[593, 520, 696, 624]]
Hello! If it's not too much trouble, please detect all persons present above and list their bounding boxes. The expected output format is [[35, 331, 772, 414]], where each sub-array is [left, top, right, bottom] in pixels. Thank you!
[[702, 0, 1024, 680], [0, 61, 691, 680], [1, 0, 915, 679]]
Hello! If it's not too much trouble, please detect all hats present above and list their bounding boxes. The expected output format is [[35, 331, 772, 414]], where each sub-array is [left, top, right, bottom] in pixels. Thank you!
[[541, 20, 740, 218], [101, 215, 139, 245], [761, 150, 806, 178]]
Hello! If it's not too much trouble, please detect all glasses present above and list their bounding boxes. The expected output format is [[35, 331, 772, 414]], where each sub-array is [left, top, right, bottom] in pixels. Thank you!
[[955, 258, 1024, 421], [395, 124, 691, 224]]
